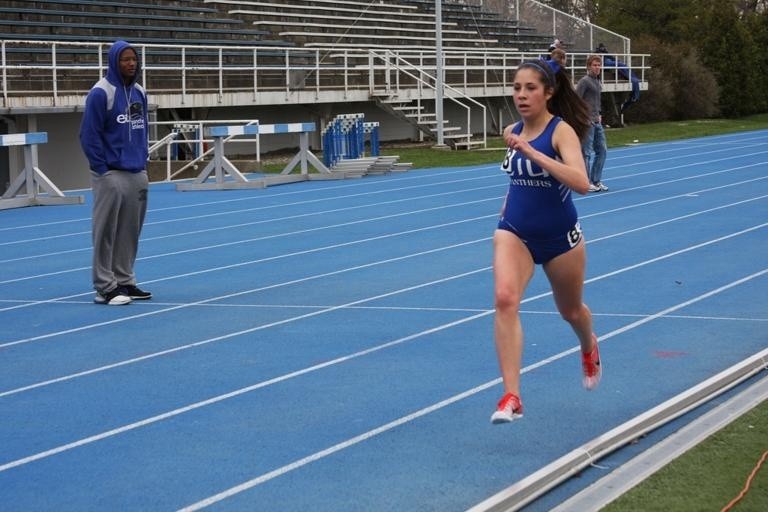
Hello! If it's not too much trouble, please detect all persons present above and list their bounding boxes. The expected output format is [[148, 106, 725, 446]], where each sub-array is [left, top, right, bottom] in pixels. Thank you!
[[595, 43, 609, 72], [545, 39, 560, 62], [544, 49, 568, 76], [481, 55, 602, 426], [568, 56, 610, 192], [78, 38, 155, 305]]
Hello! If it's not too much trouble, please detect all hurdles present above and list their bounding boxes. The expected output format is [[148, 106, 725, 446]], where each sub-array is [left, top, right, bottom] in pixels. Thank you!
[[0, 132, 84, 210], [176, 120, 346, 191], [321, 113, 413, 178]]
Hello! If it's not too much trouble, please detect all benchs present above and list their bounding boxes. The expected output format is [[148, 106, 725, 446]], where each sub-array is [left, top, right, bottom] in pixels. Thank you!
[[0, 0, 628, 96]]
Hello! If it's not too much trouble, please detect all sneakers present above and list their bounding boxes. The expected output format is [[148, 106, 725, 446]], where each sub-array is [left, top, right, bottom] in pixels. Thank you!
[[491, 392, 523, 425], [587, 181, 608, 192], [94, 286, 153, 305], [582, 332, 602, 390]]
